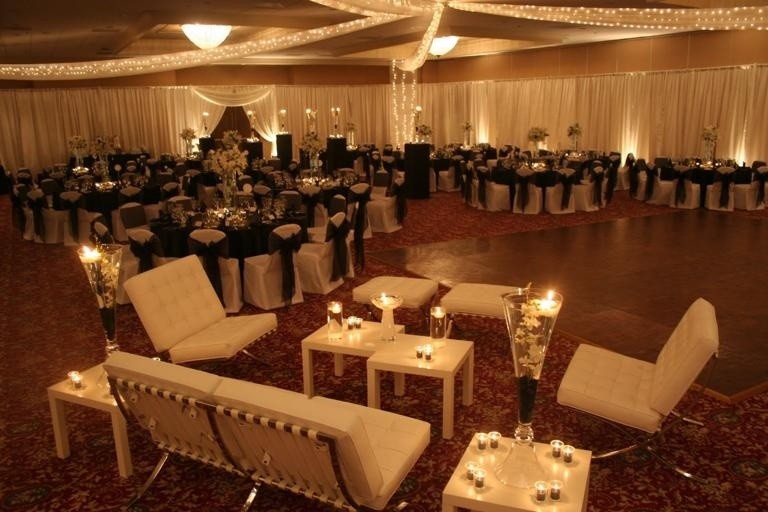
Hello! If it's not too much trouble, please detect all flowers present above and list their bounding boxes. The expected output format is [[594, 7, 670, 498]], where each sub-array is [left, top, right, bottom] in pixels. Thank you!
[[414, 124, 436, 137], [527, 128, 549, 144], [344, 121, 359, 132], [567, 124, 584, 137], [702, 126, 720, 143], [461, 120, 473, 132]]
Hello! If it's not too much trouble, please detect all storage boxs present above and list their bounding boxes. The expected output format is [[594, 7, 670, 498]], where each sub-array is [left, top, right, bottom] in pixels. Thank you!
[[101, 351, 431, 511]]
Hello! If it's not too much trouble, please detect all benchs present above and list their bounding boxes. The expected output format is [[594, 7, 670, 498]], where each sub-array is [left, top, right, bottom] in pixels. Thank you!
[[101, 351, 431, 511]]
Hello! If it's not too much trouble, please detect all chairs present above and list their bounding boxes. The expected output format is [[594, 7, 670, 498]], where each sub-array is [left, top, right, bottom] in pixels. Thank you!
[[0, 128, 405, 311], [554, 298, 719, 485], [406, 142, 767, 216], [122, 253, 279, 367]]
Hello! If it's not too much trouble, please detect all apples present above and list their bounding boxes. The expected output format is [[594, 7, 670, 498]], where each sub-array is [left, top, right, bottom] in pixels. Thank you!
[[347, 316, 362, 329], [414, 345, 432, 360], [465, 431, 575, 501], [415, 344, 433, 361], [68, 370, 83, 389]]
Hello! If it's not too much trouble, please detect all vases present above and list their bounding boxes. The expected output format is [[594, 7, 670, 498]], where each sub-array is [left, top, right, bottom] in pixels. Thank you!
[[702, 126, 720, 143], [76, 244, 123, 389], [461, 120, 473, 132], [527, 128, 549, 144], [567, 124, 584, 137], [344, 121, 359, 132], [495, 290, 563, 488], [414, 124, 436, 137]]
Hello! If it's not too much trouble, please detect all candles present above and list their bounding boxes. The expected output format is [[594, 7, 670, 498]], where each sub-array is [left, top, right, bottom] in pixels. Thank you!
[[68, 370, 83, 389], [415, 344, 433, 361], [465, 431, 575, 501], [414, 345, 432, 360], [347, 316, 362, 329]]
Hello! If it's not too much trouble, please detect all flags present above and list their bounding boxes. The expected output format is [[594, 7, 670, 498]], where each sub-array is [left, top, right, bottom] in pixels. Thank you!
[[76, 244, 123, 389], [495, 290, 563, 488]]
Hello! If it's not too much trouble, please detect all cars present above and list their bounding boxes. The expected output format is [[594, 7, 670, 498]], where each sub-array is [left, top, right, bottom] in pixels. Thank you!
[[67, 371, 83, 389], [327, 301, 344, 340], [348, 316, 362, 330], [415, 344, 433, 360], [464, 431, 575, 504], [430, 306, 447, 348]]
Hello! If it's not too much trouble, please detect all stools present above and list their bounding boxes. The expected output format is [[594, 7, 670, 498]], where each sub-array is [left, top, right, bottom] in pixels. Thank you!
[[439, 282, 520, 332], [351, 276, 439, 321]]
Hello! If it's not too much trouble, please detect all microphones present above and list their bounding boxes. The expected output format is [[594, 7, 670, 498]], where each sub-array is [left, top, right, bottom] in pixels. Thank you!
[[351, 276, 439, 321], [439, 282, 520, 332]]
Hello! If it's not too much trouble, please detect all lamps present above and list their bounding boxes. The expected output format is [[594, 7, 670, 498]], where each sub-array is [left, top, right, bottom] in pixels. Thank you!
[[554, 298, 719, 485], [122, 253, 279, 367]]
